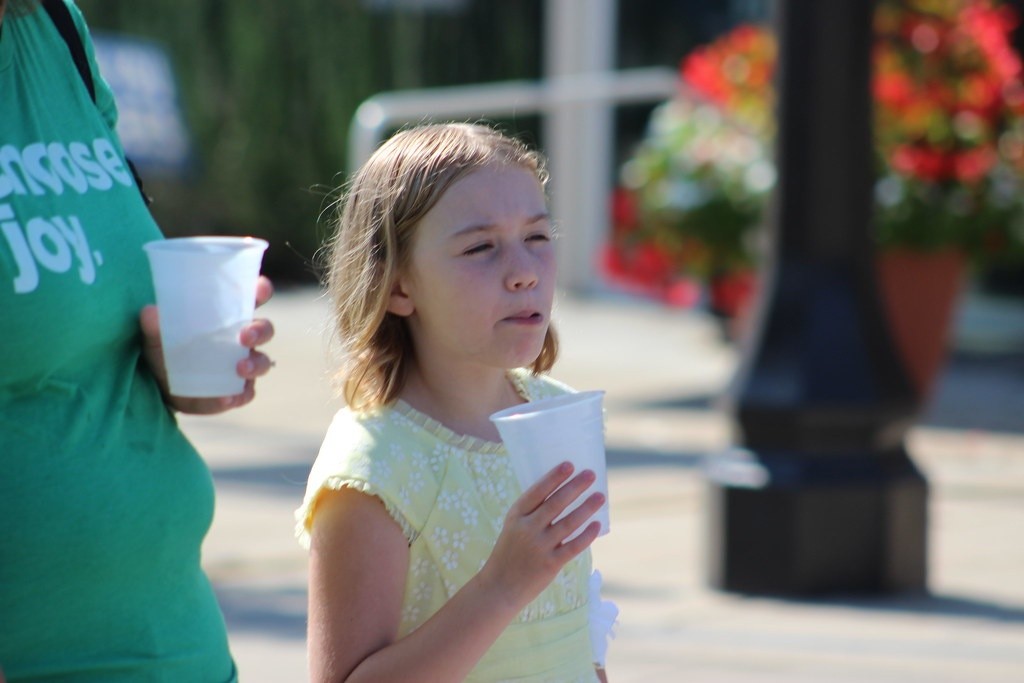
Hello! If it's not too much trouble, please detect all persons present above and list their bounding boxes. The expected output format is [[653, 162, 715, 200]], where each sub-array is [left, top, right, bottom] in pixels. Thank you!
[[294, 123, 618, 683], [0, 0, 275, 683]]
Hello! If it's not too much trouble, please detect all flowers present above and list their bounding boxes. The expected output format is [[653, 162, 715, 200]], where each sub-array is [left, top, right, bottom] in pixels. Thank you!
[[601, 2, 1024, 311]]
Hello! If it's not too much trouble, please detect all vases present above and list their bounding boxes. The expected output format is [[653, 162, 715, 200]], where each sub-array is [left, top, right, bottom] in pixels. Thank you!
[[705, 242, 966, 405]]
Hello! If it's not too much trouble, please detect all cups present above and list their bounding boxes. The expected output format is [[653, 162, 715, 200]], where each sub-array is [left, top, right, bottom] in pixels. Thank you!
[[488, 389, 610, 545], [141, 235, 270, 399]]
